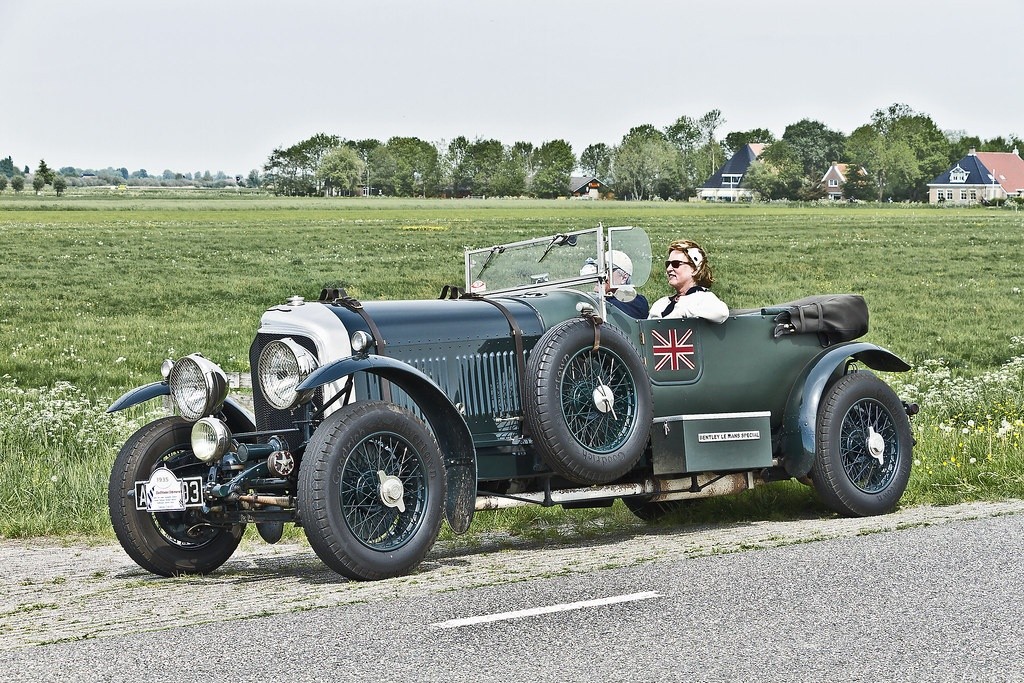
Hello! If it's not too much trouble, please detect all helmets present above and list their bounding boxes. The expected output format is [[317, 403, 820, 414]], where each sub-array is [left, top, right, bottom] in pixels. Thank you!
[[596, 249, 632, 275]]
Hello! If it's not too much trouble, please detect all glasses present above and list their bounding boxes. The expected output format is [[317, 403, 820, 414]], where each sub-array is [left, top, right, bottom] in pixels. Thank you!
[[597, 266, 619, 275], [665, 260, 693, 268]]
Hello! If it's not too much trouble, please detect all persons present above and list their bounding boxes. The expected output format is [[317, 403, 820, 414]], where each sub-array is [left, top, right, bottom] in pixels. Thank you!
[[593, 250, 650, 319], [647, 240, 729, 324]]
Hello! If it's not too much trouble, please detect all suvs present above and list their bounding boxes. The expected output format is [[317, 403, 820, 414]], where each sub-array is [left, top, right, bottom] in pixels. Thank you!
[[104, 223, 920, 583]]
